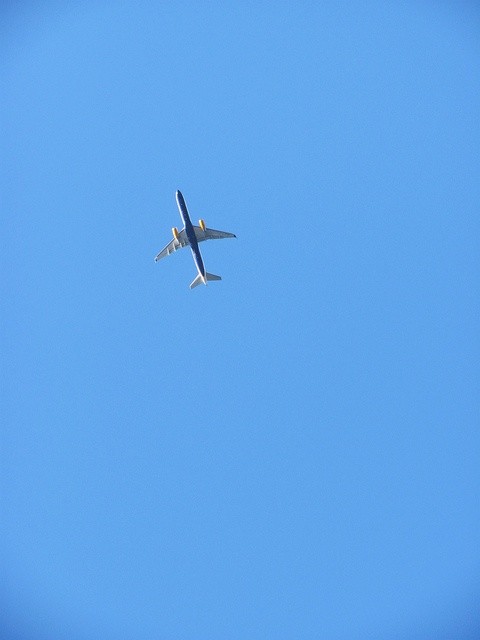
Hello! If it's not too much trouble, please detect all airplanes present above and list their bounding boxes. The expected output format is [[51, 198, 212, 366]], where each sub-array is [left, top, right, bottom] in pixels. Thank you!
[[152, 188, 238, 292]]
